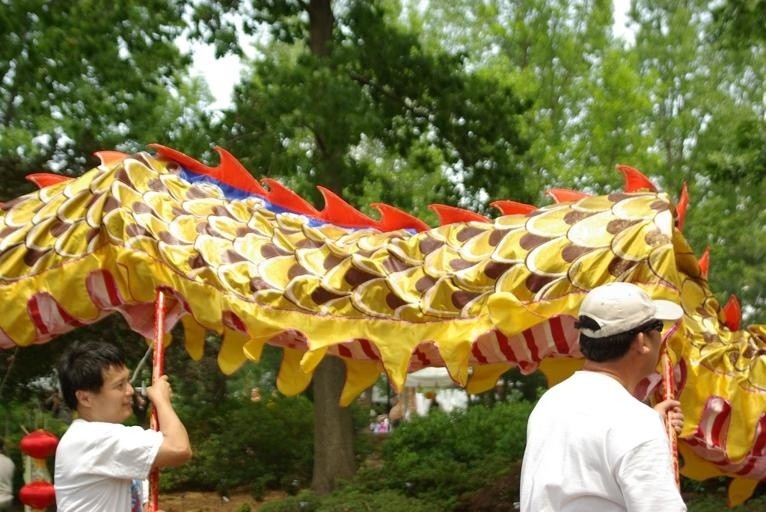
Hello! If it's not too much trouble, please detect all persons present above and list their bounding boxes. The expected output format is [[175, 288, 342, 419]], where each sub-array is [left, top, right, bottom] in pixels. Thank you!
[[0, 438, 17, 512], [370, 397, 442, 433], [249, 386, 280, 410], [52, 337, 195, 512], [517, 280, 689, 511]]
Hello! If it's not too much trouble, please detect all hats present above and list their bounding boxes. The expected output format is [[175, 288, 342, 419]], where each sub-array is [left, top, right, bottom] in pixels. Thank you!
[[576, 282, 685, 339]]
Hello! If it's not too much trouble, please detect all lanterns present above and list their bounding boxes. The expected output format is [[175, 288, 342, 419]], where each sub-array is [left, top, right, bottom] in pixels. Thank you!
[[20, 428, 58, 467], [18, 476, 56, 512]]
